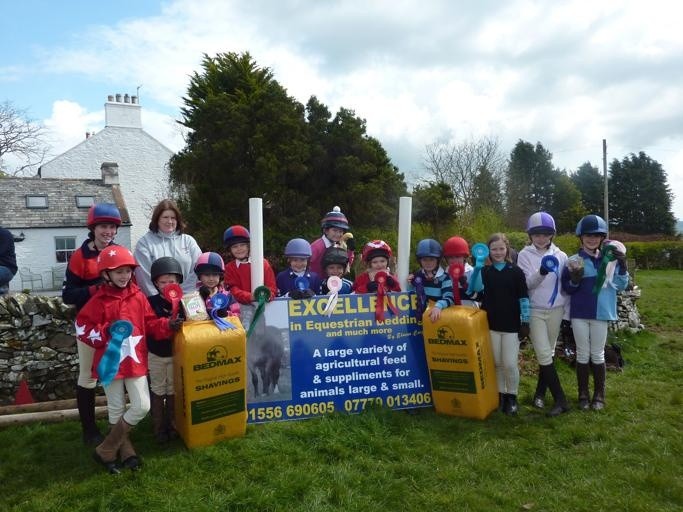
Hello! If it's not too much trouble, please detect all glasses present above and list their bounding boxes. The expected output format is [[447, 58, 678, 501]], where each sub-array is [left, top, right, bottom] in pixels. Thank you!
[[229, 243, 249, 249]]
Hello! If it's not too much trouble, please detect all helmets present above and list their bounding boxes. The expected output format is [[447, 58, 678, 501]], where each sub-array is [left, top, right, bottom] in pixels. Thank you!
[[525, 212, 556, 234], [222, 225, 250, 249], [321, 246, 349, 268], [416, 238, 442, 258], [193, 252, 225, 275], [442, 236, 470, 256], [97, 245, 140, 276], [362, 240, 392, 261], [150, 256, 184, 282], [88, 203, 122, 225], [575, 215, 608, 236], [284, 238, 312, 258], [320, 206, 350, 230]]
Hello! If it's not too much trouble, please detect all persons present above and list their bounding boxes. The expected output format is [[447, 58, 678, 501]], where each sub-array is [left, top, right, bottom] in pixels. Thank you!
[[406, 239, 454, 321], [459, 232, 529, 417], [442, 236, 476, 307], [145, 256, 182, 445], [516, 211, 574, 416], [278, 238, 321, 299], [0, 224, 18, 299], [193, 252, 240, 325], [562, 215, 628, 413], [133, 198, 204, 297], [63, 201, 122, 446], [351, 240, 401, 294], [308, 210, 353, 279], [74, 244, 183, 473], [320, 246, 353, 296], [223, 224, 276, 304]]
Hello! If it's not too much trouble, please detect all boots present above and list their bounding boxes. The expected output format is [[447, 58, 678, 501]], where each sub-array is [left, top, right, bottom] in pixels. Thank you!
[[77, 385, 180, 468], [499, 357, 606, 413]]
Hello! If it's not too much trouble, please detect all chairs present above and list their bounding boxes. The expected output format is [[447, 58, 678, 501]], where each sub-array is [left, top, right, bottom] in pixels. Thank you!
[[51, 264, 67, 290], [19, 266, 44, 293]]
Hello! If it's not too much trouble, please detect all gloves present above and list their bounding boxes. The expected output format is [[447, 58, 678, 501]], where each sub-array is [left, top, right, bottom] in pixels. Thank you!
[[169, 314, 184, 330], [609, 240, 628, 269], [303, 289, 316, 298], [289, 287, 302, 299], [518, 322, 530, 341]]
